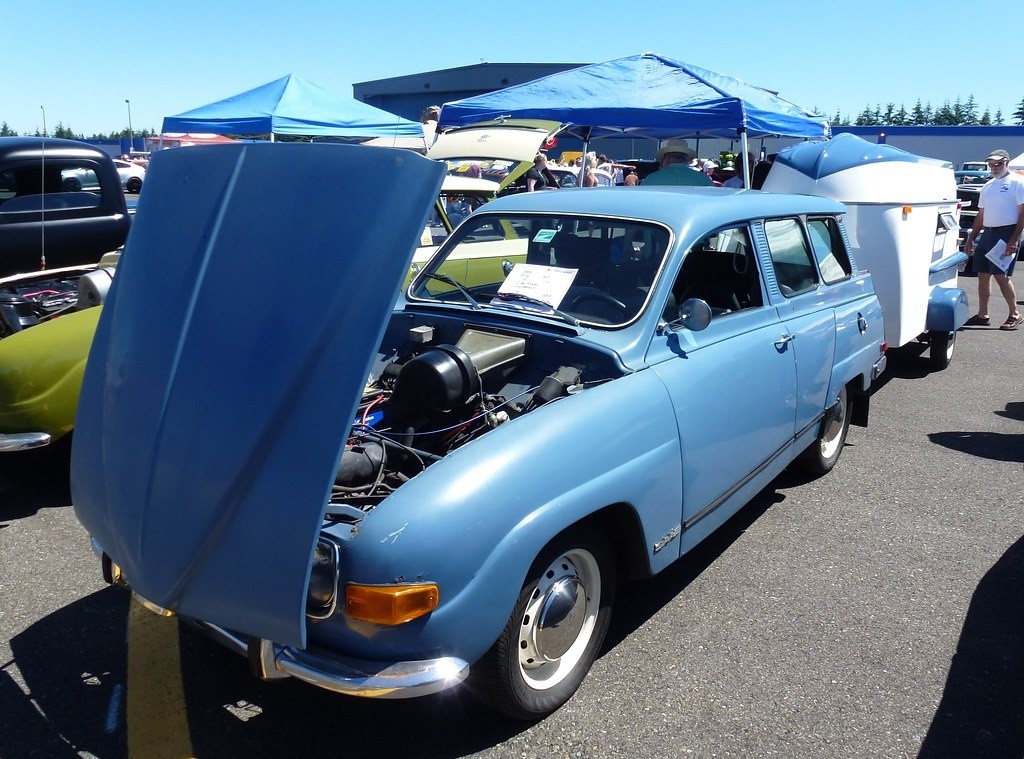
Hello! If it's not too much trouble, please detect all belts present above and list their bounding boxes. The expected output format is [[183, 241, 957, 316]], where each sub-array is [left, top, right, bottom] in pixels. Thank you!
[[984, 225, 1016, 232]]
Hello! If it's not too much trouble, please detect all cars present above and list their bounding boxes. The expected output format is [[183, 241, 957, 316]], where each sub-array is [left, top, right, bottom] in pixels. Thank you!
[[62, 142, 889, 722], [0, 244, 130, 454], [60, 159, 147, 194], [953, 160, 1024, 261], [762, 132, 972, 370], [400, 115, 660, 297]]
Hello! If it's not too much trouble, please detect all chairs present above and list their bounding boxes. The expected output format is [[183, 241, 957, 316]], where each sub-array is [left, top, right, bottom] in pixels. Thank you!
[[554, 235, 610, 291], [680, 251, 752, 311]]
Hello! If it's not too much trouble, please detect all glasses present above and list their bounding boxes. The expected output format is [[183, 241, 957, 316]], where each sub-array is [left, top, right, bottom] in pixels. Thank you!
[[988, 160, 1008, 167]]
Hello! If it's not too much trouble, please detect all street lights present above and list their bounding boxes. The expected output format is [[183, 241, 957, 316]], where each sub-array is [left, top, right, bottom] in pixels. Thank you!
[[41, 106, 47, 138], [125, 99, 133, 152]]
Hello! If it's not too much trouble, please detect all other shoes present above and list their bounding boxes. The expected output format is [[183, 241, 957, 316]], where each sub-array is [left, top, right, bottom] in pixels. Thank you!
[[999, 314, 1024, 330], [963, 315, 990, 326]]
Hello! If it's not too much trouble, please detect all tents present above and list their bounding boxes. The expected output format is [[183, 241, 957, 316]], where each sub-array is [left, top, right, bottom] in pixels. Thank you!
[[158, 72, 430, 156], [145, 130, 242, 160], [435, 50, 833, 190]]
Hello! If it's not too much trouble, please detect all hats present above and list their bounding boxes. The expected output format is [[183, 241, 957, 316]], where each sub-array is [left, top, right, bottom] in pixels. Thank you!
[[702, 161, 719, 169], [654, 139, 695, 163], [985, 149, 1010, 163]]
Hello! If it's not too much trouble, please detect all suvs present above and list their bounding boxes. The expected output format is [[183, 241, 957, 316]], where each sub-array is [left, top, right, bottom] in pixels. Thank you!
[[0, 136, 133, 281]]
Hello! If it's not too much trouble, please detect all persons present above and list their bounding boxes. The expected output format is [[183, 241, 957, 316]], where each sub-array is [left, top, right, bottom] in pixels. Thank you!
[[445, 137, 756, 242], [963, 149, 1024, 330]]
